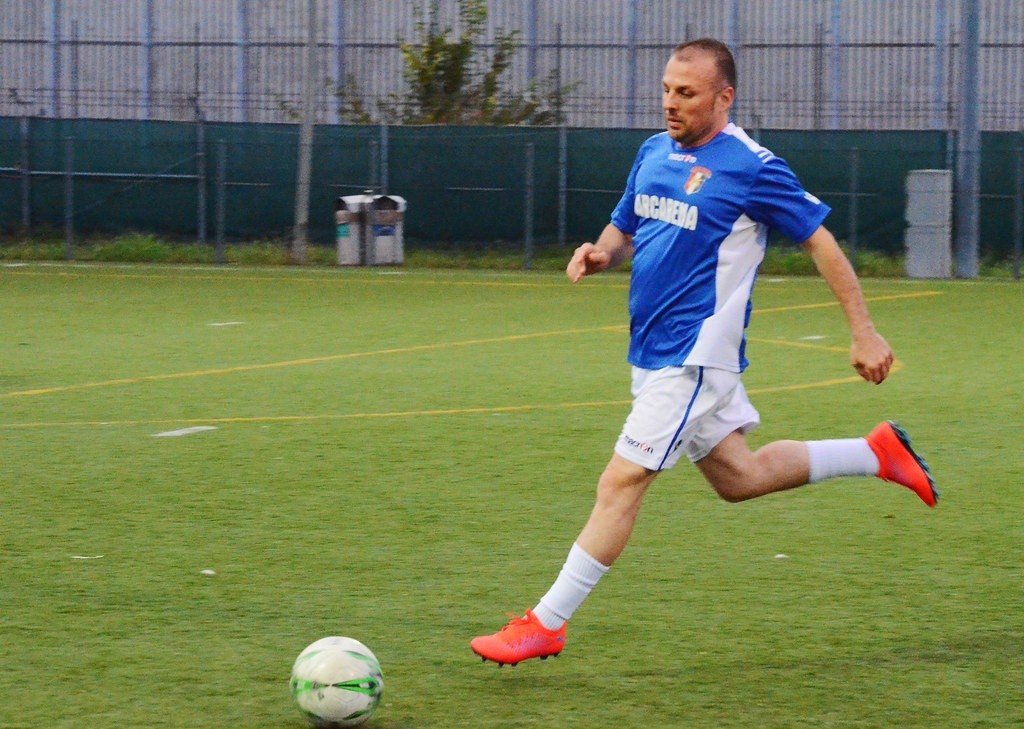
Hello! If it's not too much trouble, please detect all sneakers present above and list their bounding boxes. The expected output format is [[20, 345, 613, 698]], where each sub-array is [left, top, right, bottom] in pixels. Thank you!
[[866, 418, 941, 507], [471, 608, 566, 666]]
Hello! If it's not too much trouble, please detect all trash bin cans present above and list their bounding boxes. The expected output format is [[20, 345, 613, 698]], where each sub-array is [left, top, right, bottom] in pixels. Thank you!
[[335, 193, 408, 265]]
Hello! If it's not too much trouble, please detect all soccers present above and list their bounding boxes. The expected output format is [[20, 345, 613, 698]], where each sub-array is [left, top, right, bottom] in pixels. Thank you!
[[289, 636, 386, 729]]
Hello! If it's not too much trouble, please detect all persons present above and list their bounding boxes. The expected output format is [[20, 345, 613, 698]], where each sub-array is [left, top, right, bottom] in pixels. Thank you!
[[470, 38, 939, 668]]
